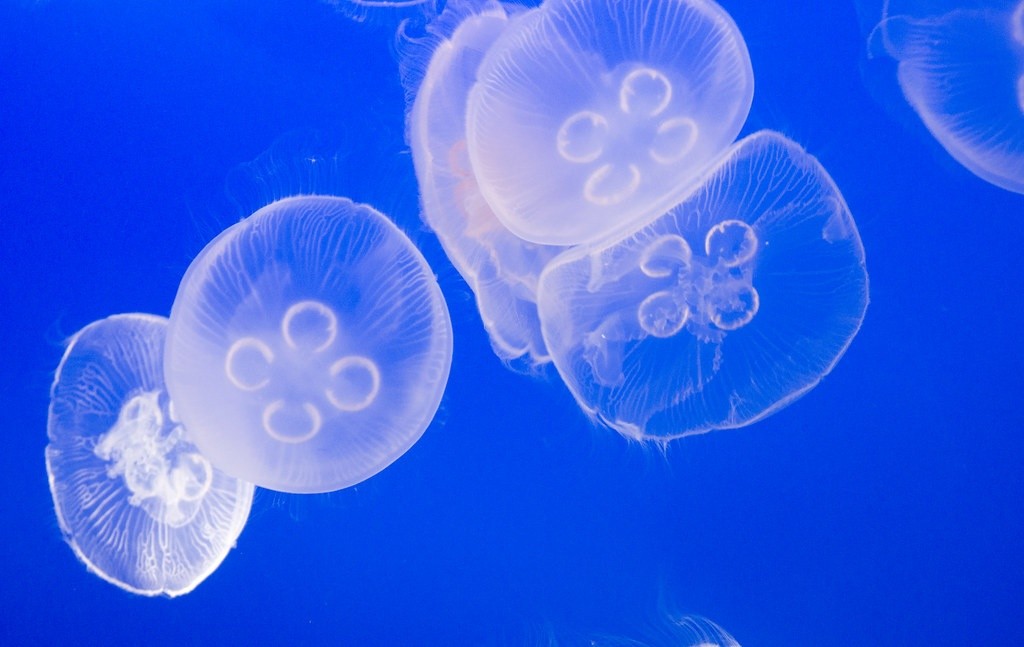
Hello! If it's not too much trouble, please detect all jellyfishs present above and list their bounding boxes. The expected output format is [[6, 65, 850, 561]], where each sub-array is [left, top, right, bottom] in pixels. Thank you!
[[42, 192, 455, 600], [864, 1, 1024, 198], [340, 0, 871, 454]]
